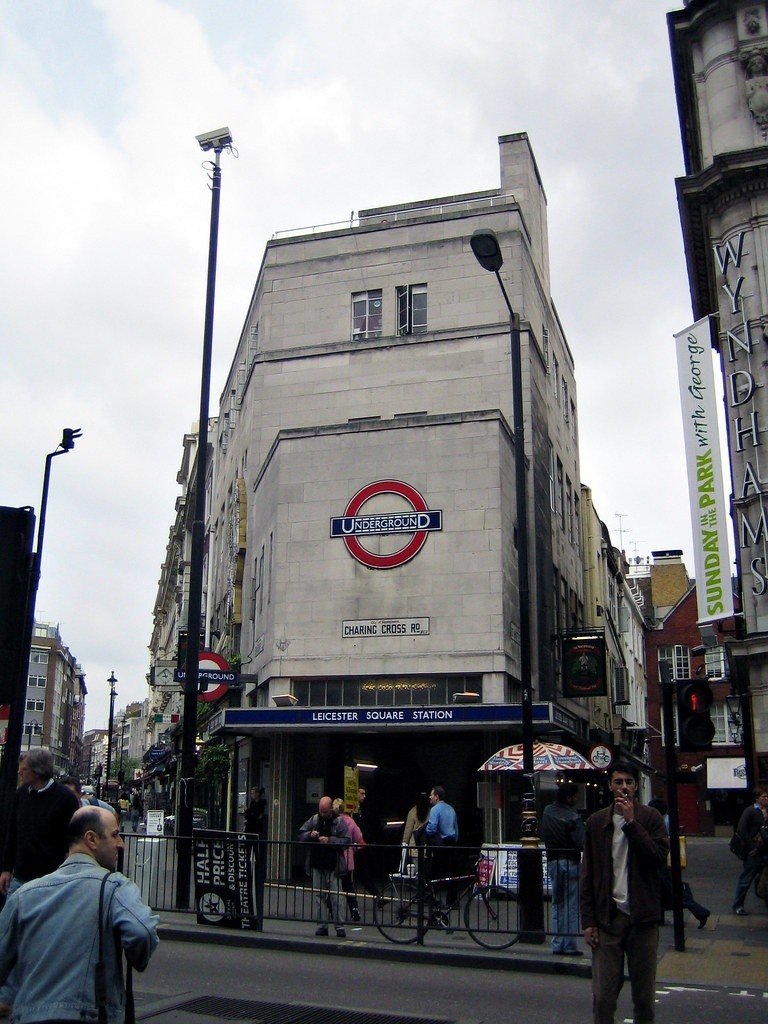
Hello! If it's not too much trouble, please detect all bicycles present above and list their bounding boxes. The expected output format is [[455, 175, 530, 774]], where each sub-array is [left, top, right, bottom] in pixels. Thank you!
[[372, 847, 524, 951]]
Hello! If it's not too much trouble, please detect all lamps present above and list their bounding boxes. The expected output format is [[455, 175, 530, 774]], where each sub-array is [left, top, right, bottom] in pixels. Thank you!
[[468, 228, 517, 318], [692, 643, 725, 675]]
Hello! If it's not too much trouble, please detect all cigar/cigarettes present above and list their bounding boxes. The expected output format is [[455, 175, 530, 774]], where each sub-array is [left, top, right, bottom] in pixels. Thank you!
[[625, 794, 628, 796]]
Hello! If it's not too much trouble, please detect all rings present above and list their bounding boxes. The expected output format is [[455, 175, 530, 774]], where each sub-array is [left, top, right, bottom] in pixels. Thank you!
[[624, 800, 628, 804]]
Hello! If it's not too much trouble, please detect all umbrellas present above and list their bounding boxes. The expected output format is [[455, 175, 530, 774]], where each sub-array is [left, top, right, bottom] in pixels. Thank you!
[[476, 737, 596, 773]]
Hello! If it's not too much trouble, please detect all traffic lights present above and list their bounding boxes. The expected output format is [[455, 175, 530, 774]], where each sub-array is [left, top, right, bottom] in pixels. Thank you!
[[675, 676, 716, 754]]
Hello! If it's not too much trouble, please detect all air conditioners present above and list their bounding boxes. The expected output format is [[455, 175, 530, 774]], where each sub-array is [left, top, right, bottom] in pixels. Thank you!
[[614, 667, 630, 706]]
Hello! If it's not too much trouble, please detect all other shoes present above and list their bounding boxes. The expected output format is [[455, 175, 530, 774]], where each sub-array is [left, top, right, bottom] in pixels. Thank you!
[[337, 929, 346, 937], [439, 903, 459, 910], [316, 928, 329, 935], [698, 911, 711, 928], [735, 907, 749, 916], [352, 908, 361, 921], [553, 951, 585, 956]]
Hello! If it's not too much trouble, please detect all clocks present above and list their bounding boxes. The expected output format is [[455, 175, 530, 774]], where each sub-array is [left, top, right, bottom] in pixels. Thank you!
[[157, 668, 174, 685]]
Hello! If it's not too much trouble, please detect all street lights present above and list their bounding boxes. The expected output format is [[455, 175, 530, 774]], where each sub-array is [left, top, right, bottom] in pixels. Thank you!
[[171, 126, 235, 910], [101, 670, 119, 805], [469, 224, 549, 946], [26, 718, 44, 751]]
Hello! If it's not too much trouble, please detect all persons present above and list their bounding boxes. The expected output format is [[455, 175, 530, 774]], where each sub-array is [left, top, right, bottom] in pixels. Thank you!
[[0, 804, 161, 1024], [649, 797, 711, 929], [399, 783, 462, 909], [542, 781, 586, 957], [579, 763, 668, 1024], [130, 796, 145, 833], [296, 795, 367, 938], [350, 787, 394, 909], [0, 749, 120, 906], [728, 786, 768, 916], [242, 784, 268, 881]]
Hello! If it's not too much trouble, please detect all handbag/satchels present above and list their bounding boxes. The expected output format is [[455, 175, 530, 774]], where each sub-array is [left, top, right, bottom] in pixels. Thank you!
[[729, 833, 750, 861], [755, 866, 768, 899]]
